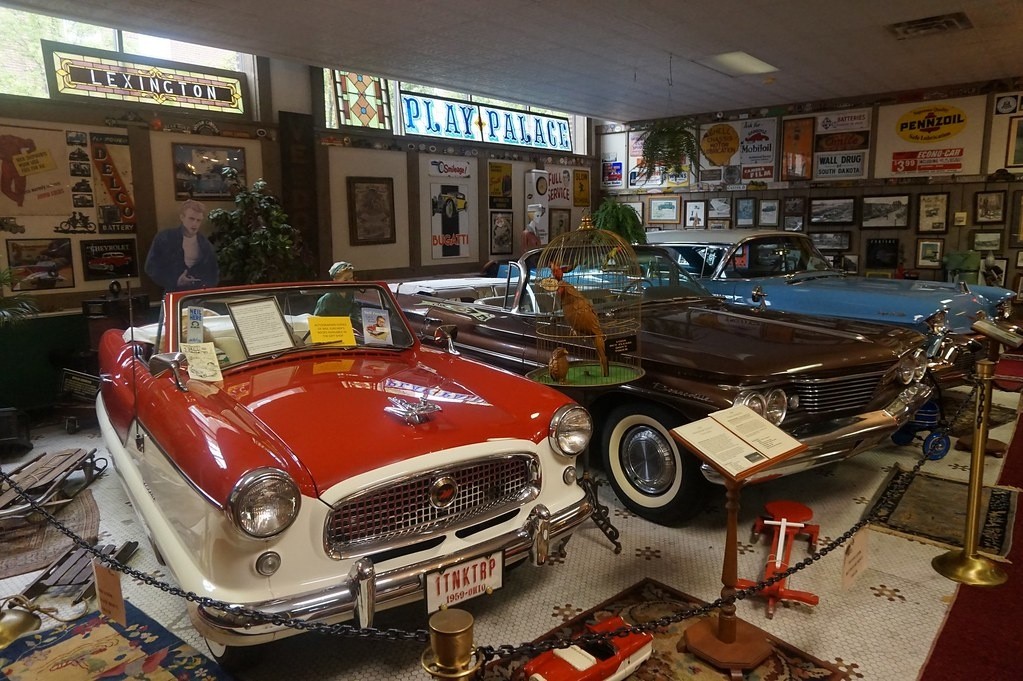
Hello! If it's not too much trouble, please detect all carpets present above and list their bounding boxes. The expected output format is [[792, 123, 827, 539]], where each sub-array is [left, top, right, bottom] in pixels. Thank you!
[[0, 601, 228, 681], [858, 462, 1023, 563], [468, 576, 852, 681], [931, 397, 1018, 437], [0, 488, 99, 580]]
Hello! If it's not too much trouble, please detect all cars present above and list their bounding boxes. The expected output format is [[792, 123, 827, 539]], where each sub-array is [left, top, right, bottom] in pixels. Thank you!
[[339, 241, 933, 527], [94, 280, 596, 669], [522, 615, 654, 681], [494, 229, 1023, 388]]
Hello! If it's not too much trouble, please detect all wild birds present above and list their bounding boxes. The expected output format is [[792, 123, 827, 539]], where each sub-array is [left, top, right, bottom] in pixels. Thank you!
[[557, 280, 609, 377], [549, 347, 571, 384], [547, 260, 580, 283]]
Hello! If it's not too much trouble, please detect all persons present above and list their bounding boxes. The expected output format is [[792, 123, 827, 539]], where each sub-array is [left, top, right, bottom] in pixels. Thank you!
[[519, 204, 542, 268], [144, 199, 219, 291], [366, 316, 385, 337], [314, 261, 363, 334]]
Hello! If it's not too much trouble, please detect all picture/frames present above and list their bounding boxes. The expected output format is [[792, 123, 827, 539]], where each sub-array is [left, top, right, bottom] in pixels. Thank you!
[[781, 118, 815, 183], [1004, 115, 1023, 168], [548, 208, 571, 245], [489, 211, 513, 255], [171, 142, 249, 202], [617, 190, 1023, 305], [345, 176, 397, 246]]
